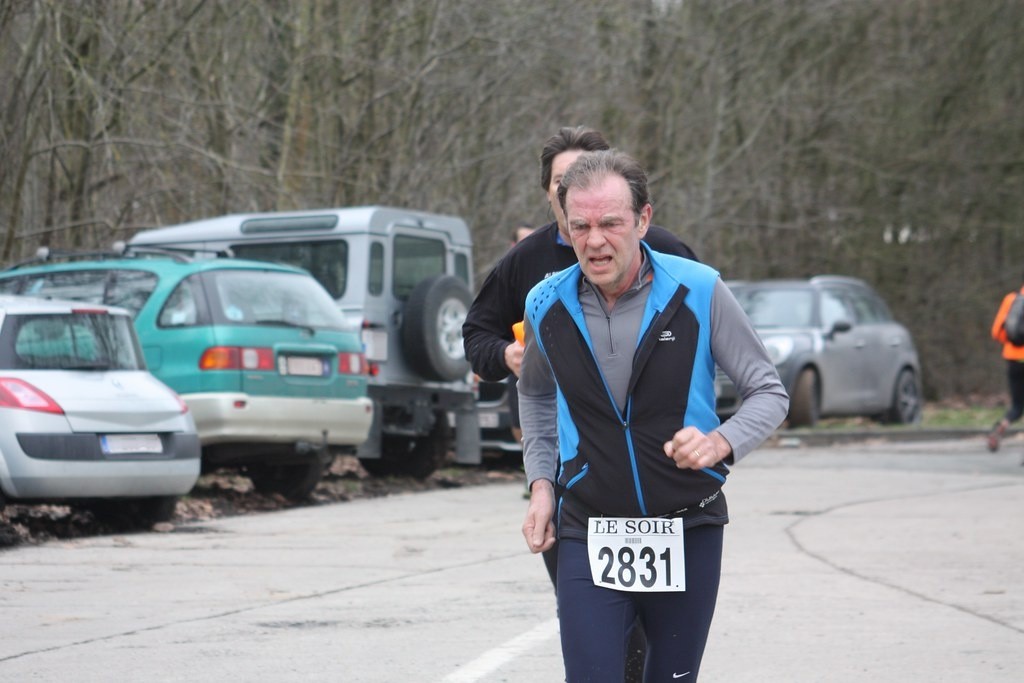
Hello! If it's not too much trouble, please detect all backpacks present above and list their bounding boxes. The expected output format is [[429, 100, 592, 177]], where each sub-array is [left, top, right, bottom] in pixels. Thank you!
[[1002, 290, 1024, 346]]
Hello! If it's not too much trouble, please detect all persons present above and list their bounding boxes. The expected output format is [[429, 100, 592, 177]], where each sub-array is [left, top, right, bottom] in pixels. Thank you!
[[517, 150, 790, 682], [463, 127, 699, 683], [988, 285, 1024, 453]]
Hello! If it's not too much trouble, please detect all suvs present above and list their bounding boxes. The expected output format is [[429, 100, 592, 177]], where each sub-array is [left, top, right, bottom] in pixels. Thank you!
[[0, 244, 378, 527], [124, 205, 533, 482], [712, 275, 921, 428]]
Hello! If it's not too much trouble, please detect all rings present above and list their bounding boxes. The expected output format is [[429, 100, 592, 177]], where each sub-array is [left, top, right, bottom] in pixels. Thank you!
[[694, 450, 701, 458]]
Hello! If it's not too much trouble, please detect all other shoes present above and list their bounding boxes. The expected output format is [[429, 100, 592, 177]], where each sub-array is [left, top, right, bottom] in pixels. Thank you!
[[988, 421, 1002, 454]]
